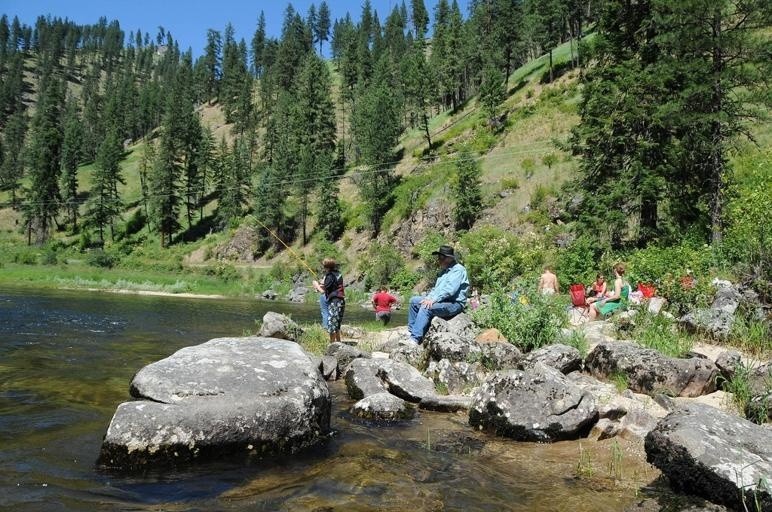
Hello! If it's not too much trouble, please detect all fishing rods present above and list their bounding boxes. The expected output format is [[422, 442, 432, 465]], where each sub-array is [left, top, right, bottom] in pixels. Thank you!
[[247, 213, 320, 281]]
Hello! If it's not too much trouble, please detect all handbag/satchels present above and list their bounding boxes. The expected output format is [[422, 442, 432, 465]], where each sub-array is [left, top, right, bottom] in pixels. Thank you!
[[570, 283, 585, 306]]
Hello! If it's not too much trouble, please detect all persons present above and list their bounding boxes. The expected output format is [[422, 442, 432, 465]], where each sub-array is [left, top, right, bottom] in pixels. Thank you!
[[585, 265, 629, 319], [319, 261, 340, 330], [312, 258, 345, 343], [371, 286, 396, 327], [408, 246, 470, 344], [537, 266, 560, 296]]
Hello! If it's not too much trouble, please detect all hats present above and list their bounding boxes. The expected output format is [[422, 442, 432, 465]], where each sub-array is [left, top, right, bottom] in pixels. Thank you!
[[432, 245, 456, 258]]
